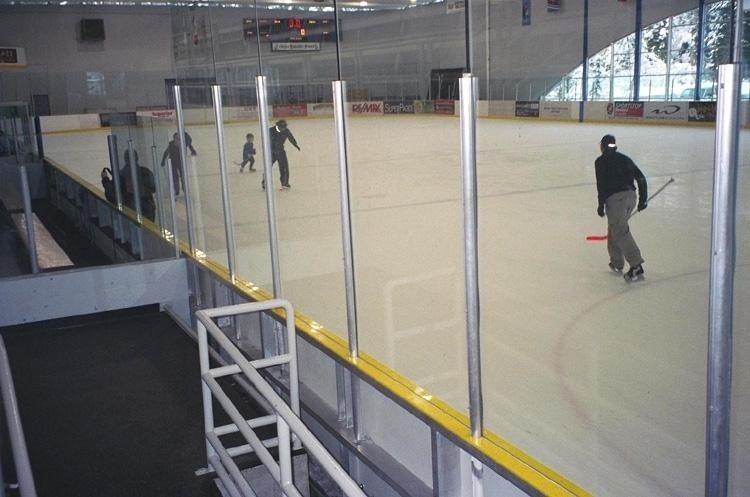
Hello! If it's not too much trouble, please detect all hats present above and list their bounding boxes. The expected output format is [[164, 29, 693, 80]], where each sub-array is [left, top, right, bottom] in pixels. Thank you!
[[276, 120, 287, 128], [601, 135, 617, 151]]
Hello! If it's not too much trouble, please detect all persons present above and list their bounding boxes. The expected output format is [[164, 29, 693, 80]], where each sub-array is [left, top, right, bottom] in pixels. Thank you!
[[161, 130, 199, 202], [99, 146, 159, 226], [591, 131, 650, 281], [237, 131, 259, 173], [259, 118, 301, 190]]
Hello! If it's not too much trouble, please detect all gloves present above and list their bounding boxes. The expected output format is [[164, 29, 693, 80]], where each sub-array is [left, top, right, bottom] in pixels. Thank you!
[[598, 205, 604, 217], [638, 200, 647, 212]]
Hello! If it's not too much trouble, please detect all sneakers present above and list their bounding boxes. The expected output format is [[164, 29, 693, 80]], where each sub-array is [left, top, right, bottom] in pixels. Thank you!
[[624, 265, 644, 280], [609, 263, 623, 270], [281, 183, 291, 188]]
